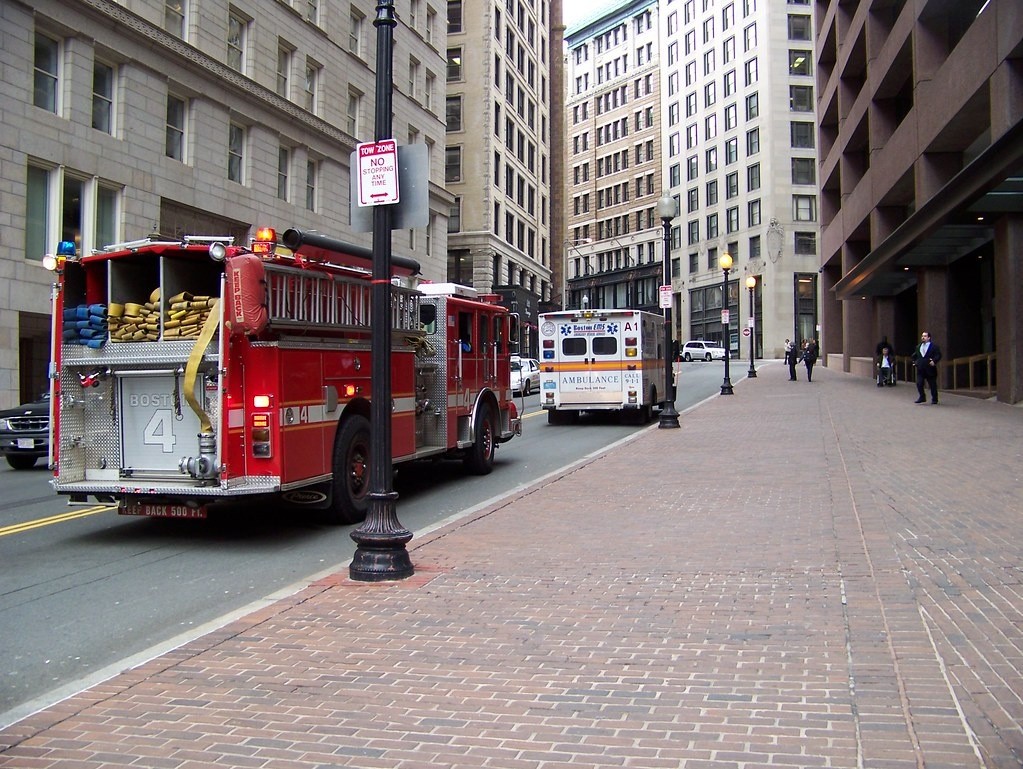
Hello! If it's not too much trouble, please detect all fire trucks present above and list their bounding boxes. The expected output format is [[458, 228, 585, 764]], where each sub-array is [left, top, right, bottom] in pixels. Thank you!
[[47, 227, 527, 524]]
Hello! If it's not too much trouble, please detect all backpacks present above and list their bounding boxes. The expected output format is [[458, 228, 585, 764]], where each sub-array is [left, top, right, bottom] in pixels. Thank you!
[[803, 349, 812, 362]]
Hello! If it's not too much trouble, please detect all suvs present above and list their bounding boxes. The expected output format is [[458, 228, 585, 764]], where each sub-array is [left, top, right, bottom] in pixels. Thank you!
[[0, 385, 54, 471]]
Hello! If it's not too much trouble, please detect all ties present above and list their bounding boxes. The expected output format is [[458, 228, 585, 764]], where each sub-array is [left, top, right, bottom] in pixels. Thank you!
[[923, 342, 926, 357]]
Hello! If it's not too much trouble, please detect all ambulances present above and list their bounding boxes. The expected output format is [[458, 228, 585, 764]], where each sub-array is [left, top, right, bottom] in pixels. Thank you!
[[537, 308, 683, 427]]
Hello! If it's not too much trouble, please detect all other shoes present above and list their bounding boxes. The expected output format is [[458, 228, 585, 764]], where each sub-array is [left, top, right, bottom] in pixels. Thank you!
[[931, 400, 938, 405], [914, 398, 926, 403]]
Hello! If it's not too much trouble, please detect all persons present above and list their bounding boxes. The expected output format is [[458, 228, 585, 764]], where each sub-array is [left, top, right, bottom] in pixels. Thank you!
[[798, 337, 818, 382], [784, 339, 798, 381], [876, 336, 896, 386], [462, 340, 472, 352], [912, 332, 942, 404]]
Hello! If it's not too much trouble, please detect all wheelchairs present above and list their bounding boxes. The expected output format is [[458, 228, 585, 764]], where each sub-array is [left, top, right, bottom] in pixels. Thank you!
[[876, 363, 895, 387]]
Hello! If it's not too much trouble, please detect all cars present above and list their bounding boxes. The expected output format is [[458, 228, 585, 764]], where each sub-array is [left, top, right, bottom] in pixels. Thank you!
[[681, 340, 732, 362], [511, 355, 540, 397]]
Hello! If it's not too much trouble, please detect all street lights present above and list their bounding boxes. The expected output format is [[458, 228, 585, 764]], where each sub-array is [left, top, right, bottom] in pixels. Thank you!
[[582, 294, 589, 309], [746, 274, 757, 378], [656, 188, 681, 428], [718, 250, 735, 395]]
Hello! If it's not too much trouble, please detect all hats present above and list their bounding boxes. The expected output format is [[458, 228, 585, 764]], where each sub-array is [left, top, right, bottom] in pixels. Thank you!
[[804, 343, 811, 348]]
